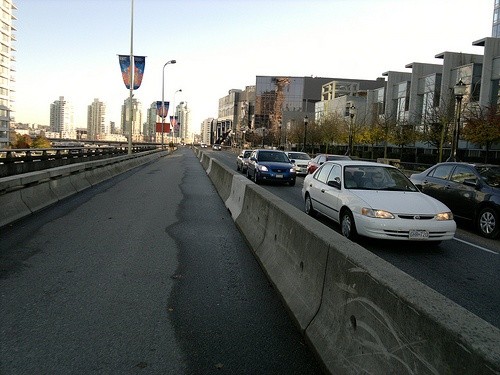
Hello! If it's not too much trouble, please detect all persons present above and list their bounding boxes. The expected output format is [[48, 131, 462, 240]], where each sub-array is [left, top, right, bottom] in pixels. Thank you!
[[1, 150, 27, 163], [169, 141, 173, 151], [365, 173, 386, 189]]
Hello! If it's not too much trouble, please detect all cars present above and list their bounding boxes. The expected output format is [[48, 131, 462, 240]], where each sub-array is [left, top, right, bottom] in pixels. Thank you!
[[201, 144, 207, 148], [302, 160, 456, 244], [285, 151, 312, 176], [410, 162, 500, 236], [306, 154, 352, 175], [181, 143, 186, 146], [247, 149, 296, 186], [236, 149, 253, 173], [212, 144, 221, 151]]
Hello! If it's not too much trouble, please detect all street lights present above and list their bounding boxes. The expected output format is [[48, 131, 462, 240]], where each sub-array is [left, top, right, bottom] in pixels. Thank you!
[[303, 114, 308, 151], [161, 59, 176, 151], [172, 89, 182, 148], [454, 77, 466, 164], [261, 124, 266, 149], [232, 131, 235, 150], [349, 103, 355, 158], [279, 120, 282, 148], [243, 126, 246, 149]]
